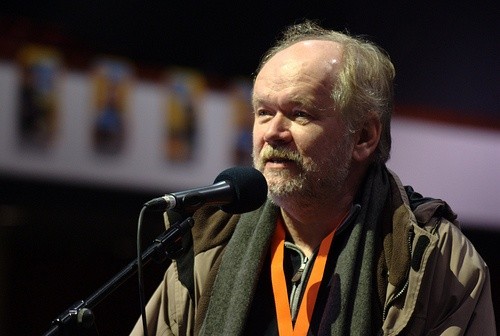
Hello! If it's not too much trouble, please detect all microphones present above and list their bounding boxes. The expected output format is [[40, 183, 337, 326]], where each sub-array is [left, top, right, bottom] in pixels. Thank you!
[[144, 166, 268, 215]]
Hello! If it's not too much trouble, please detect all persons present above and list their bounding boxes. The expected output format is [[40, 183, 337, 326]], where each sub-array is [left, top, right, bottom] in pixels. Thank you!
[[128, 22, 498, 336]]
